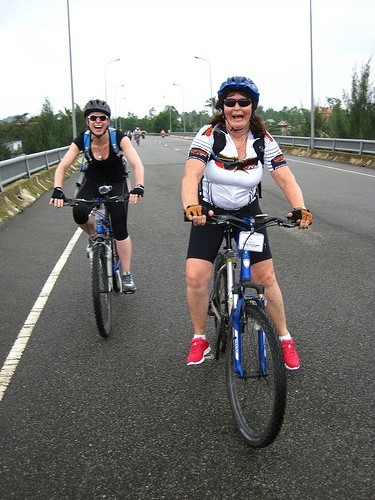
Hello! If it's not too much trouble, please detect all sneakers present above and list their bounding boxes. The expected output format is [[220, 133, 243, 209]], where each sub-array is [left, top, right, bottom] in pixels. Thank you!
[[280, 338, 301, 370], [121, 272, 136, 292], [186, 338, 211, 366], [86, 235, 94, 253]]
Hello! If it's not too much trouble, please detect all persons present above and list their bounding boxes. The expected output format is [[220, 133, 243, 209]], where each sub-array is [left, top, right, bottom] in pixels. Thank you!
[[180, 76, 313, 370], [49, 99, 144, 294], [160, 130, 166, 136], [124, 127, 146, 142]]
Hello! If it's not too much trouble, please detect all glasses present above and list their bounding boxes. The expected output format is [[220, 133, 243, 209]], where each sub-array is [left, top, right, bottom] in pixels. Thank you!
[[88, 116, 107, 121], [223, 99, 251, 107]]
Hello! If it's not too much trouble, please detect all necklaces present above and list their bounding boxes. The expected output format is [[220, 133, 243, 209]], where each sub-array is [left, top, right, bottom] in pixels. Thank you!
[[236, 134, 248, 150], [94, 148, 105, 159]]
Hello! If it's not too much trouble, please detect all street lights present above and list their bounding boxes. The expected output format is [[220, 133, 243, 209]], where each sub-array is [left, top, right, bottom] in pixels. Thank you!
[[104, 58, 120, 104], [173, 83, 186, 132], [162, 96, 172, 131], [119, 98, 127, 132], [196, 56, 214, 120], [115, 84, 124, 129]]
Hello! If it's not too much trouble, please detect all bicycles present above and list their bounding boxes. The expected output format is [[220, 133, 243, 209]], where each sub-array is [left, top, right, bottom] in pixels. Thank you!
[[52, 185, 137, 339], [194, 209, 308, 449], [141, 132, 146, 139], [127, 133, 140, 145], [161, 134, 164, 138]]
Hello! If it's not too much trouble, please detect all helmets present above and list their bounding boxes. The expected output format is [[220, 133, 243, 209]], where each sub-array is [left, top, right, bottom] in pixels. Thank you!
[[218, 76, 259, 103], [83, 99, 110, 118]]
